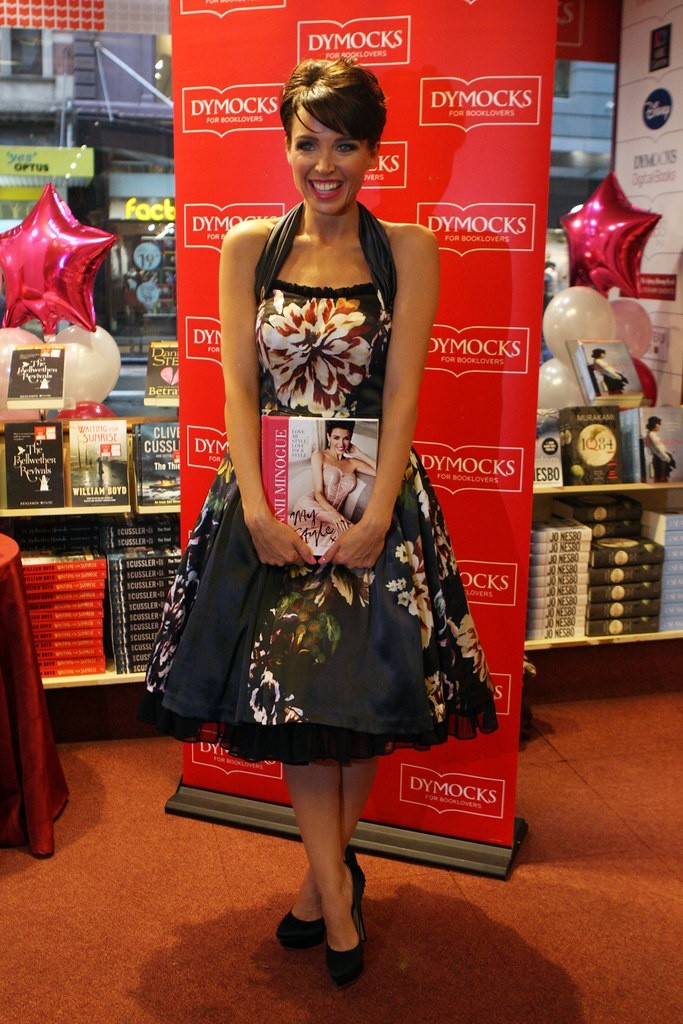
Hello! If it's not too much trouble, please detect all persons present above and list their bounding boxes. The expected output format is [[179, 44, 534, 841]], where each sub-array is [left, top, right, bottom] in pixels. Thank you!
[[646, 416, 672, 482], [292, 420, 377, 547], [592, 348, 625, 394], [545, 262, 558, 307], [137, 60, 499, 986]]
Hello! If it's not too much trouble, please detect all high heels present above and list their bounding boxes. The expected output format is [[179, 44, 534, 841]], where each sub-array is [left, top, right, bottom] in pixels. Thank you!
[[274, 845, 366, 951], [326, 861, 367, 988]]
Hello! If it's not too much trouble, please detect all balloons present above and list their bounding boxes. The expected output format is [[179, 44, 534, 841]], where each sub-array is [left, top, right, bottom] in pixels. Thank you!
[[0, 329, 121, 509], [0, 182, 116, 333], [560, 171, 662, 299], [537, 286, 658, 408]]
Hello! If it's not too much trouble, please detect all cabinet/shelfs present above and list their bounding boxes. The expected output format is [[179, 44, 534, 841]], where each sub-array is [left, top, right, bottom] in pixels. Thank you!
[[0, 480, 683, 680]]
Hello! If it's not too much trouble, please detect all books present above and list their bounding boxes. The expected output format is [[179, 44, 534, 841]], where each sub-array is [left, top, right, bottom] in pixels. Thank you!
[[0, 515, 183, 678], [3, 422, 65, 510], [69, 420, 129, 506], [527, 494, 683, 641], [144, 342, 180, 407], [6, 344, 67, 409], [567, 338, 644, 406], [132, 421, 180, 506], [534, 405, 683, 486], [262, 416, 378, 556]]
